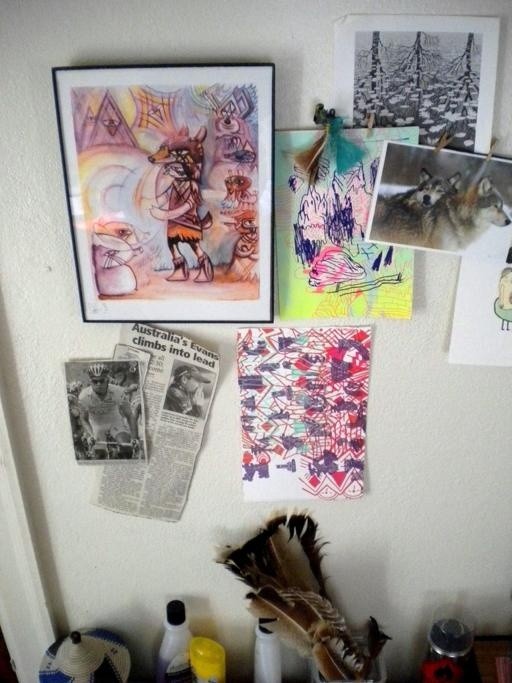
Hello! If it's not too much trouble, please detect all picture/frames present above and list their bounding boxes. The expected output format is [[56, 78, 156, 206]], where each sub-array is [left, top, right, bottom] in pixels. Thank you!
[[52, 63, 276, 323]]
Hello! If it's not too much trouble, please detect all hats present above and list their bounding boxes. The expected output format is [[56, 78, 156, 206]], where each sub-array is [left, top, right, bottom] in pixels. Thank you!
[[174, 364, 211, 384]]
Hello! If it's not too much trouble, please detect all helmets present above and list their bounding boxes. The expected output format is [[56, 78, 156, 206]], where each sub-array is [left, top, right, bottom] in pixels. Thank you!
[[88, 363, 110, 377]]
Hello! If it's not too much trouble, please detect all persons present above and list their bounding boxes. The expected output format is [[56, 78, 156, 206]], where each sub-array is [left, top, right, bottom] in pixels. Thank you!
[[163, 364, 211, 419], [67, 362, 146, 460]]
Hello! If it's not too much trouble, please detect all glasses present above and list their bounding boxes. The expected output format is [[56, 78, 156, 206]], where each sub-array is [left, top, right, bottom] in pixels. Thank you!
[[92, 379, 106, 384]]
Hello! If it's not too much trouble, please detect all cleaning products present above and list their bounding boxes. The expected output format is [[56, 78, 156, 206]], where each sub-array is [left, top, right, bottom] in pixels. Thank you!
[[158, 600, 193, 682], [190, 638, 226, 683], [254, 626, 282, 683]]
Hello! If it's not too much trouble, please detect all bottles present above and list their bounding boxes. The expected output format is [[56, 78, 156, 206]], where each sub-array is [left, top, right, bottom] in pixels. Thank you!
[[416, 618, 484, 683], [154, 599, 226, 683], [251, 615, 284, 683]]
